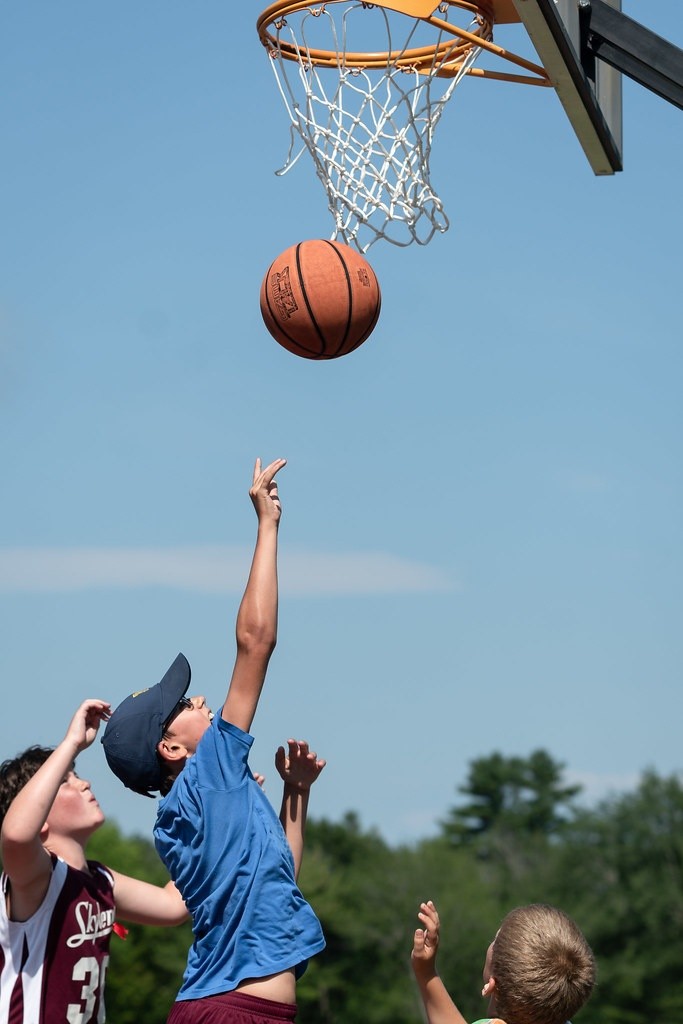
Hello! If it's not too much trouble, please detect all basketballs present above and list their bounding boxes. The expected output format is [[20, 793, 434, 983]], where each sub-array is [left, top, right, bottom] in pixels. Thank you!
[[258, 241, 379, 358]]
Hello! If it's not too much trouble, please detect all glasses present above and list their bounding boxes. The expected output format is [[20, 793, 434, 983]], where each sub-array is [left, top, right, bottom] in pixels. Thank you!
[[162, 697, 193, 733]]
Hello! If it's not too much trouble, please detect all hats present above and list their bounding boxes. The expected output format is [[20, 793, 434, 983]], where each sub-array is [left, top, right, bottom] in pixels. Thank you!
[[101, 653, 192, 798]]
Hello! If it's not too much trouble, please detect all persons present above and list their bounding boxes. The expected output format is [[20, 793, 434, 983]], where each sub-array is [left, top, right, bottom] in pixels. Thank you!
[[0, 698, 327, 1024], [96, 456, 328, 1024], [409, 899, 598, 1024]]
